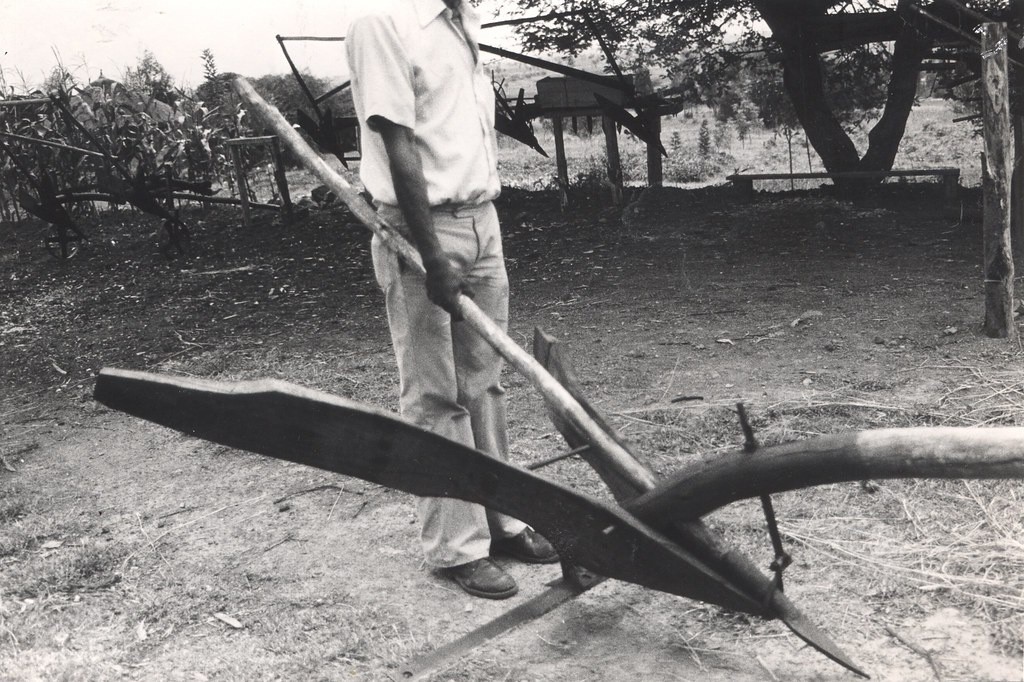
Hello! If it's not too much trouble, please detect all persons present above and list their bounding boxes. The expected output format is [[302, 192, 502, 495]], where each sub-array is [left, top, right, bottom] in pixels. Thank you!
[[345, 0, 560, 598], [716, 86, 740, 123]]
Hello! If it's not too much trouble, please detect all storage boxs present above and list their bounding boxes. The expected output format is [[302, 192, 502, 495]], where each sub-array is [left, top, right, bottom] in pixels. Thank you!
[[536, 69, 654, 109]]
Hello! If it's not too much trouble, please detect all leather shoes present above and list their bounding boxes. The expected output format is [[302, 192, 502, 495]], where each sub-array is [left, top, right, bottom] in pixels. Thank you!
[[492, 527, 560, 563], [441, 557, 518, 599]]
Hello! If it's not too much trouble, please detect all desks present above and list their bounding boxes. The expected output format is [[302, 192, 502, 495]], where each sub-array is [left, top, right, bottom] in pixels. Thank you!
[[530, 98, 683, 207]]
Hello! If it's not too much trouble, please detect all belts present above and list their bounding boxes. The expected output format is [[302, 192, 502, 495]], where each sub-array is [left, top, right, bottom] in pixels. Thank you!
[[432, 203, 476, 213]]
